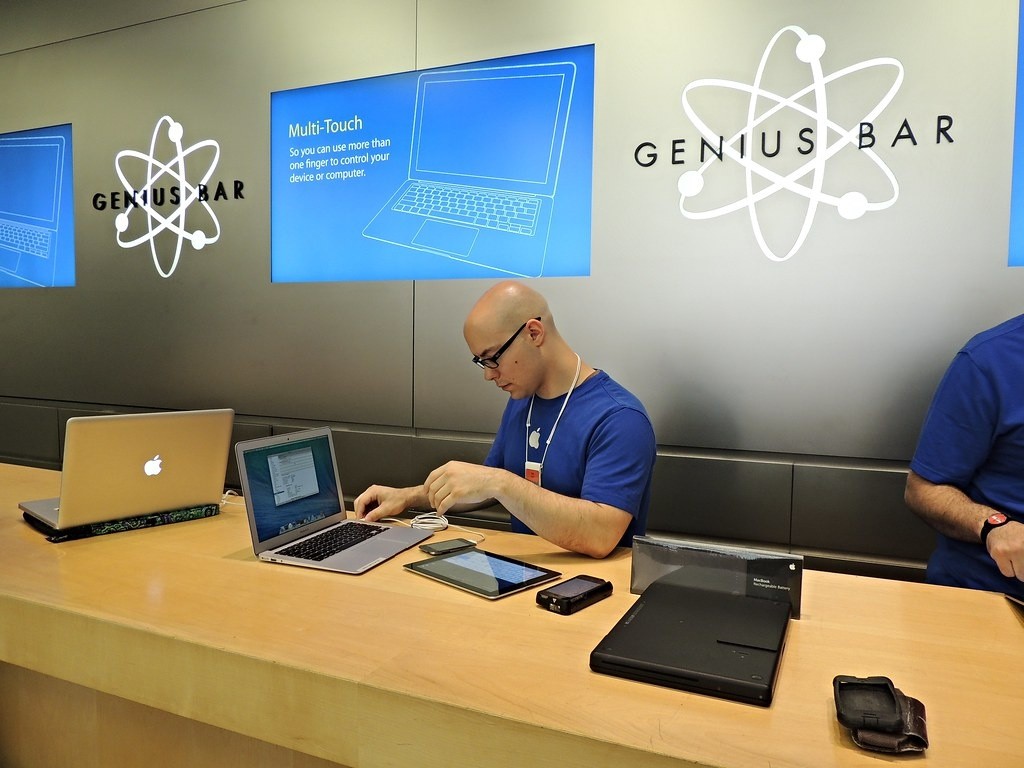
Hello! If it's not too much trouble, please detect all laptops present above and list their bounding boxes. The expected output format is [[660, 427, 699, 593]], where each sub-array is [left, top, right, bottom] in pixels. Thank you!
[[17, 408, 236, 530], [589, 580, 794, 707], [234, 426, 433, 575]]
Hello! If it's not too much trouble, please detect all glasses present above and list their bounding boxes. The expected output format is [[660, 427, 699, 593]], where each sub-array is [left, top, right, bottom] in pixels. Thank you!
[[472, 316, 542, 369]]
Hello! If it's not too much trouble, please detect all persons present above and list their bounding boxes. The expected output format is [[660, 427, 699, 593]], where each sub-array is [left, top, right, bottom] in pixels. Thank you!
[[904, 314, 1024, 603], [354, 280, 656, 560]]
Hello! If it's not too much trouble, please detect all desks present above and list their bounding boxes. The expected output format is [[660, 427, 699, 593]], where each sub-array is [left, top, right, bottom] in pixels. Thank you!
[[0, 460, 1024, 768]]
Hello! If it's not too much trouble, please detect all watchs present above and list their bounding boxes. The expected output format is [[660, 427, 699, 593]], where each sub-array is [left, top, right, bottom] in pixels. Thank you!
[[980, 511, 1013, 553]]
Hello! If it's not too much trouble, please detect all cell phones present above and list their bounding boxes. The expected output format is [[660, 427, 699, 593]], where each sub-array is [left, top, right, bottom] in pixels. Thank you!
[[419, 538, 477, 555], [536, 574, 612, 616]]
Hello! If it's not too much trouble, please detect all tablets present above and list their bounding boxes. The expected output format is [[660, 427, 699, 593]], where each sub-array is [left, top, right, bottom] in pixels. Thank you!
[[402, 547, 563, 599]]
